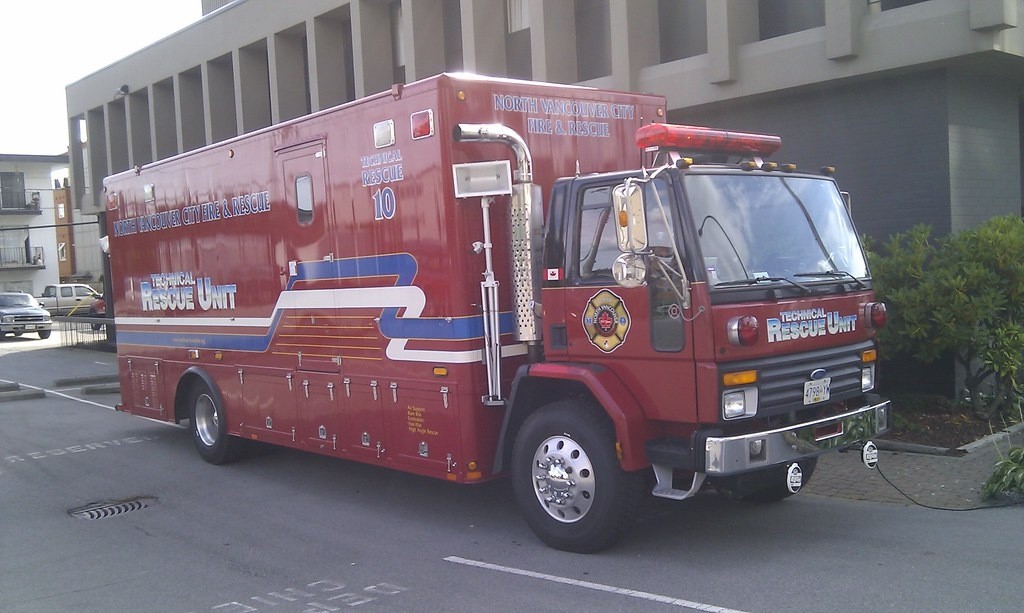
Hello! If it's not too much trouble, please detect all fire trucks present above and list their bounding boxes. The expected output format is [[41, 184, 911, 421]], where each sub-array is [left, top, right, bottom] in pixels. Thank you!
[[103, 70, 893, 555]]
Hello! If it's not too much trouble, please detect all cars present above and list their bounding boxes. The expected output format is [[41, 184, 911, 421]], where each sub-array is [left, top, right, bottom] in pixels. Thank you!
[[88, 292, 106, 331], [0, 291, 52, 340]]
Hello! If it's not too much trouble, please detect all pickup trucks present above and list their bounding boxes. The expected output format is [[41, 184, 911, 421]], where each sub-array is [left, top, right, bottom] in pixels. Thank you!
[[34, 283, 103, 318]]
